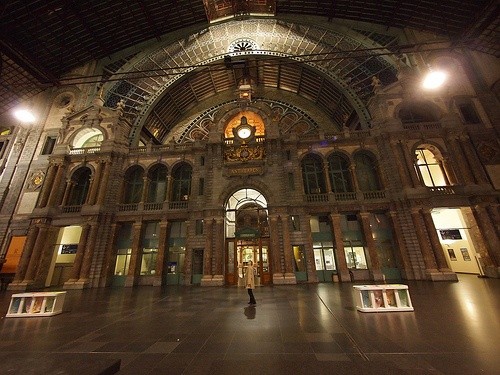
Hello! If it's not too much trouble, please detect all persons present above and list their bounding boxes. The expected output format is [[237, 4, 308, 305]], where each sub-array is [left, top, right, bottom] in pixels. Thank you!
[[245, 260, 256, 304]]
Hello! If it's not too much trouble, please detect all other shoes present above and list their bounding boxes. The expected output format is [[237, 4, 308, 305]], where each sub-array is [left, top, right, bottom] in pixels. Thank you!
[[248, 303, 255, 307]]
